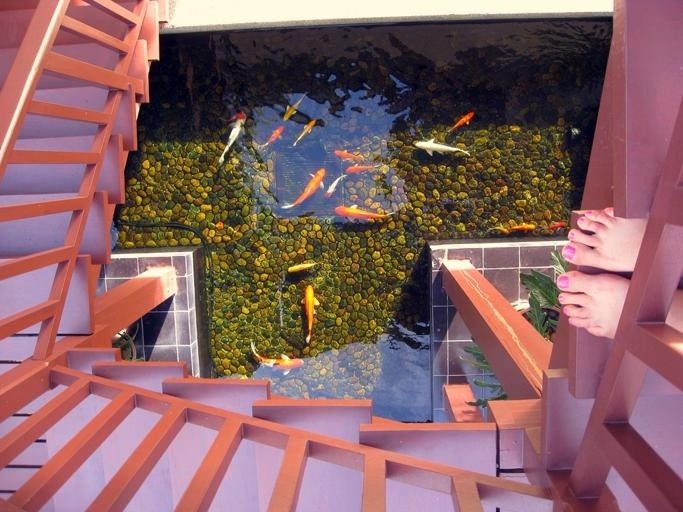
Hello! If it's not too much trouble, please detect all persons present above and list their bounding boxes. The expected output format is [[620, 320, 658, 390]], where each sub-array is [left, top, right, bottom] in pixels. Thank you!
[[557, 210, 683, 339]]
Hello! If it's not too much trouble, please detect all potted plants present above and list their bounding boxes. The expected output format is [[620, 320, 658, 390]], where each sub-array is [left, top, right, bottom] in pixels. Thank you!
[[464, 250, 573, 408]]
